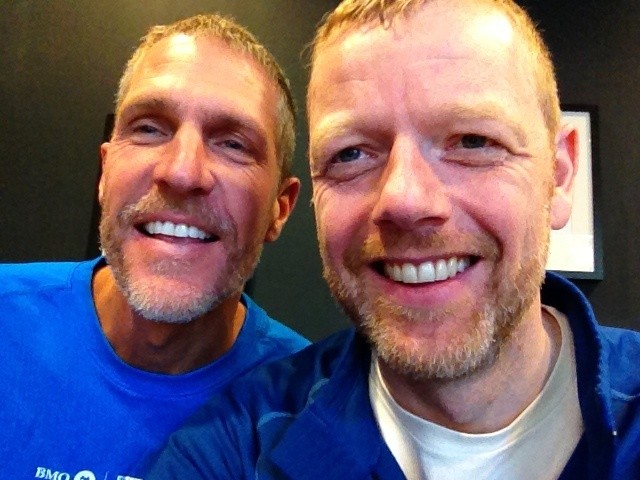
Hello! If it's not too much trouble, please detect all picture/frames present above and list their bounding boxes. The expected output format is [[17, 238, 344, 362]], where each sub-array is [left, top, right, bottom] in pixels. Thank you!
[[544, 103, 607, 281]]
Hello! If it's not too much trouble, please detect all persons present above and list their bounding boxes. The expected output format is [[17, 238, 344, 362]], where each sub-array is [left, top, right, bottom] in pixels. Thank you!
[[145, 0, 639, 480], [0, 14, 318, 480]]
